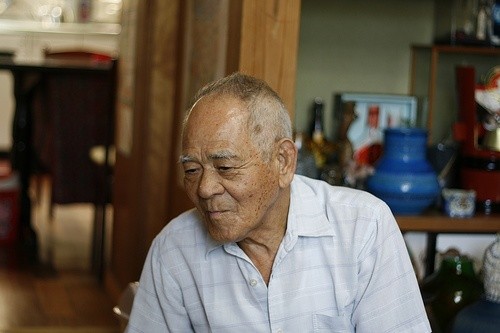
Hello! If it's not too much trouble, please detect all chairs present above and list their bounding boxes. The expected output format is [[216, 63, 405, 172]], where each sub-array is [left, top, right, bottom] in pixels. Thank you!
[[12, 45, 121, 275]]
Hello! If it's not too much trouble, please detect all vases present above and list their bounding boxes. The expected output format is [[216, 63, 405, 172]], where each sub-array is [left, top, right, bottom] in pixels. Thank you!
[[365, 128, 442, 215]]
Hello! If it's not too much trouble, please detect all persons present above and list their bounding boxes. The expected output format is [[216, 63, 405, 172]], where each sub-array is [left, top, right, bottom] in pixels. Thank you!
[[125, 72, 432, 333]]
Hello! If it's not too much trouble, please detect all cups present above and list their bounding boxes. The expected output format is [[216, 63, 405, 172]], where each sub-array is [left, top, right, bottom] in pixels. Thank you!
[[443, 188, 477, 217]]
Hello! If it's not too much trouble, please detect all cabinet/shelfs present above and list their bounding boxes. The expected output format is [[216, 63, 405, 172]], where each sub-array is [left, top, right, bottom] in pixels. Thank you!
[[409, 43, 500, 147]]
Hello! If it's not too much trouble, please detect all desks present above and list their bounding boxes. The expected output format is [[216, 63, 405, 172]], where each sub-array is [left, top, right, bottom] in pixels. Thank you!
[[0, 58, 118, 256], [393, 203, 500, 280]]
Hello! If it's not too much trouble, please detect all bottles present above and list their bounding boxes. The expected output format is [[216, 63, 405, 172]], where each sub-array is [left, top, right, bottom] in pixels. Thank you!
[[421, 241, 500, 333], [291, 96, 354, 188], [365, 128, 441, 216]]
